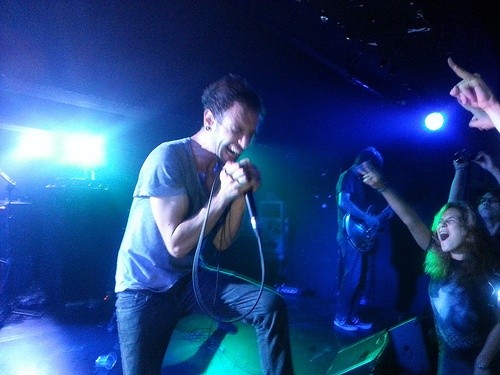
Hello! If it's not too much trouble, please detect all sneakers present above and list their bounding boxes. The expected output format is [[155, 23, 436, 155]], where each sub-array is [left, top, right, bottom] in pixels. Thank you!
[[334, 317, 360, 331], [350, 317, 372, 329]]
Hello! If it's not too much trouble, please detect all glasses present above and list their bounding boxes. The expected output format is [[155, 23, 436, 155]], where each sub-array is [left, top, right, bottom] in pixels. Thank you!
[[479, 197, 500, 203]]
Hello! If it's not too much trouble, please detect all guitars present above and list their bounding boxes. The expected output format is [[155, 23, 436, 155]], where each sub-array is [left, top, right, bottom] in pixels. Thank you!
[[341, 204, 396, 253]]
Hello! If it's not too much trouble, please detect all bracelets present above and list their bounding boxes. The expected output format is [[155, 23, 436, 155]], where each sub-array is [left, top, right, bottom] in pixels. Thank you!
[[478, 361, 489, 369]]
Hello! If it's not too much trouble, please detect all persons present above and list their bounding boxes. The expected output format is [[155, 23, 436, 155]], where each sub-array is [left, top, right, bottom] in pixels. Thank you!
[[333, 148, 382, 330], [357, 58, 500, 375], [114, 76, 296, 375]]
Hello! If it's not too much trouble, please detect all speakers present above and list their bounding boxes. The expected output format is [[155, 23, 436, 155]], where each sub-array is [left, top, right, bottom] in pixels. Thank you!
[[325, 330, 393, 375]]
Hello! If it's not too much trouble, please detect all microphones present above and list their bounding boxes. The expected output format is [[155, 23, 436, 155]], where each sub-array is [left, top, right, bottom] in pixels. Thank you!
[[244, 189, 259, 232]]
